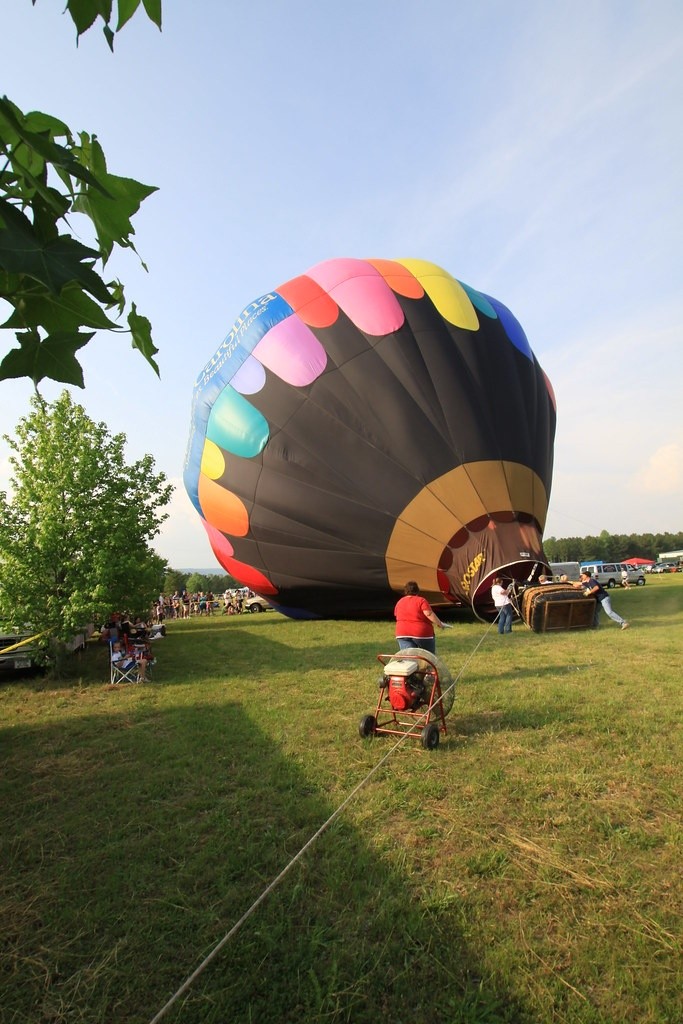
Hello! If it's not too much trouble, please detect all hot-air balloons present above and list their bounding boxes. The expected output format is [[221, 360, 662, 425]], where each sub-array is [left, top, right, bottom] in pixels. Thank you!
[[183, 257, 599, 632]]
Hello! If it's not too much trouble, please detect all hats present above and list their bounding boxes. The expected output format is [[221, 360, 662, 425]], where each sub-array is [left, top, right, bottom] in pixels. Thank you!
[[581, 571, 591, 577]]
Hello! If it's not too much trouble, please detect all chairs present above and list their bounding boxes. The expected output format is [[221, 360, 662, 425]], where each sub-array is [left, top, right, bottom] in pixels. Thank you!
[[110, 638, 140, 685], [121, 622, 147, 642]]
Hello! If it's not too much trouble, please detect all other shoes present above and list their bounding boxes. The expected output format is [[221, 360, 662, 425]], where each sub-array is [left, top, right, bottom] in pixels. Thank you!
[[622, 623, 630, 630], [140, 677, 151, 682]]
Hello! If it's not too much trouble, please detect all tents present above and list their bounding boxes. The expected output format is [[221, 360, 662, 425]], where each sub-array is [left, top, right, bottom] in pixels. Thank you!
[[623, 557, 655, 569]]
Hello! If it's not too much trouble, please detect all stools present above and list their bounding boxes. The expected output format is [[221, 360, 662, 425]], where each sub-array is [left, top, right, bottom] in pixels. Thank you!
[[137, 656, 157, 679]]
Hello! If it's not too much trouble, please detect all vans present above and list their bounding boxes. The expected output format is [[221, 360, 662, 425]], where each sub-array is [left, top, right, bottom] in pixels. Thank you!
[[0, 620, 85, 679], [224, 589, 247, 599], [580, 563, 646, 589]]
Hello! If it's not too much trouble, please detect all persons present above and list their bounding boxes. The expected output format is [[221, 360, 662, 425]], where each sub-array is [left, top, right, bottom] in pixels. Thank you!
[[120, 615, 146, 639], [490, 577, 513, 634], [133, 617, 147, 630], [558, 574, 567, 582], [539, 575, 554, 584], [569, 571, 629, 630], [112, 641, 151, 682], [150, 588, 255, 624], [393, 581, 454, 656], [621, 569, 631, 589]]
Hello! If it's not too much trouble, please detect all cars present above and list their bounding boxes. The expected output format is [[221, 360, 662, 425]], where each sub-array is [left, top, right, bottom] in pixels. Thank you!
[[245, 595, 273, 613], [644, 562, 682, 573]]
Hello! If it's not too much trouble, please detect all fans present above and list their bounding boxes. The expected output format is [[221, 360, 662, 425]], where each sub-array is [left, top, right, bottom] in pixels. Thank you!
[[358, 648, 456, 750]]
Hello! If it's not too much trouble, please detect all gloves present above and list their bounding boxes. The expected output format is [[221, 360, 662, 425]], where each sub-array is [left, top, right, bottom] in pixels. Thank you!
[[583, 591, 591, 597], [441, 622, 452, 630]]
